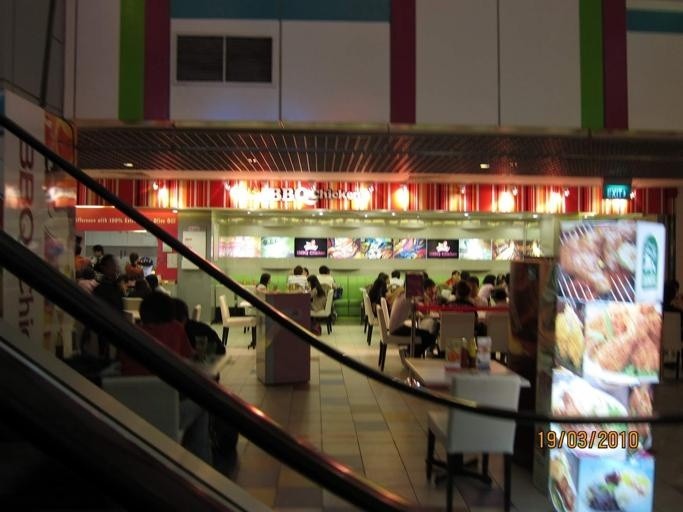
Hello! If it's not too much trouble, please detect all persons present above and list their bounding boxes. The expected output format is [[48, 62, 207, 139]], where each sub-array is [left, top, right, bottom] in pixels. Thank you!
[[368, 268, 510, 358], [256, 265, 338, 312], [74, 235, 226, 429]]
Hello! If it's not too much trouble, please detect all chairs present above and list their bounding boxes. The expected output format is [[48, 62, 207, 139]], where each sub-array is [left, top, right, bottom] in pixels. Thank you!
[[121, 283, 531, 512], [662, 311, 683, 379]]
